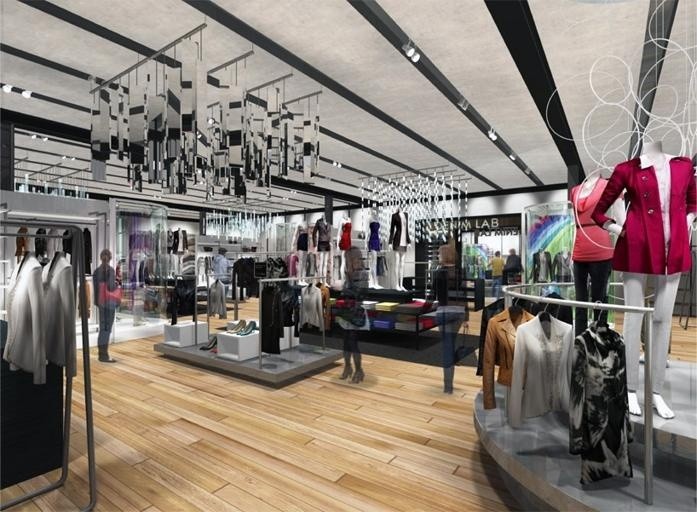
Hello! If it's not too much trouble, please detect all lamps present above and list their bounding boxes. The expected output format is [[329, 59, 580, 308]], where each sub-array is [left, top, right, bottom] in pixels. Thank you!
[[399, 36, 420, 67], [84, 1, 324, 204], [486, 126, 496, 143], [358, 163, 472, 244], [456, 93, 469, 111]]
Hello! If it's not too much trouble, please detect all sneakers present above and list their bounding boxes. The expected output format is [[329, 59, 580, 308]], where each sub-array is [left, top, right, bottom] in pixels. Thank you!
[[99, 357, 117, 362], [339, 367, 364, 383]]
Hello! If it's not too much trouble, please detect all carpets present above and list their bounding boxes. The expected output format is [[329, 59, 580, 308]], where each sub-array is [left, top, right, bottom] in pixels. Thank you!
[[298, 324, 480, 368], [118, 303, 241, 321]]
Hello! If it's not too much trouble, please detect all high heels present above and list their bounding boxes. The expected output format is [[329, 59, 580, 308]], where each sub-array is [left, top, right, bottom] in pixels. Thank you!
[[200, 336, 217, 350], [227, 319, 257, 336]]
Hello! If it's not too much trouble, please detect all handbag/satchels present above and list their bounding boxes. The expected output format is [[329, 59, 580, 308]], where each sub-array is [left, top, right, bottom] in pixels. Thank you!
[[453, 325, 478, 367], [98, 281, 124, 308]]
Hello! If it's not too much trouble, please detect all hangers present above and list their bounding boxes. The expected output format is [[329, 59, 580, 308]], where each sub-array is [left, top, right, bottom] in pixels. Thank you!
[[507, 292, 521, 313], [19, 233, 31, 253], [536, 296, 549, 320], [51, 235, 62, 253], [591, 299, 606, 332]]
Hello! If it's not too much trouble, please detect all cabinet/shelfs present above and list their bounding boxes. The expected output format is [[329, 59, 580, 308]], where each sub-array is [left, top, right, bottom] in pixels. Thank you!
[[181, 236, 258, 301], [402, 276, 485, 313], [322, 297, 443, 346]]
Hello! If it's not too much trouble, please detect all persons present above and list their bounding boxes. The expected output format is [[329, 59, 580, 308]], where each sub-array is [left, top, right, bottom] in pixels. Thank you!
[[368, 218, 385, 290], [212, 247, 228, 280], [486, 250, 504, 300], [590, 139, 696, 419], [337, 216, 352, 281], [312, 216, 331, 288], [337, 247, 368, 384], [503, 249, 521, 284], [529, 248, 552, 283], [552, 247, 574, 282], [92, 249, 120, 362], [291, 222, 309, 287], [389, 207, 411, 292], [569, 175, 624, 340], [418, 244, 470, 394]]
